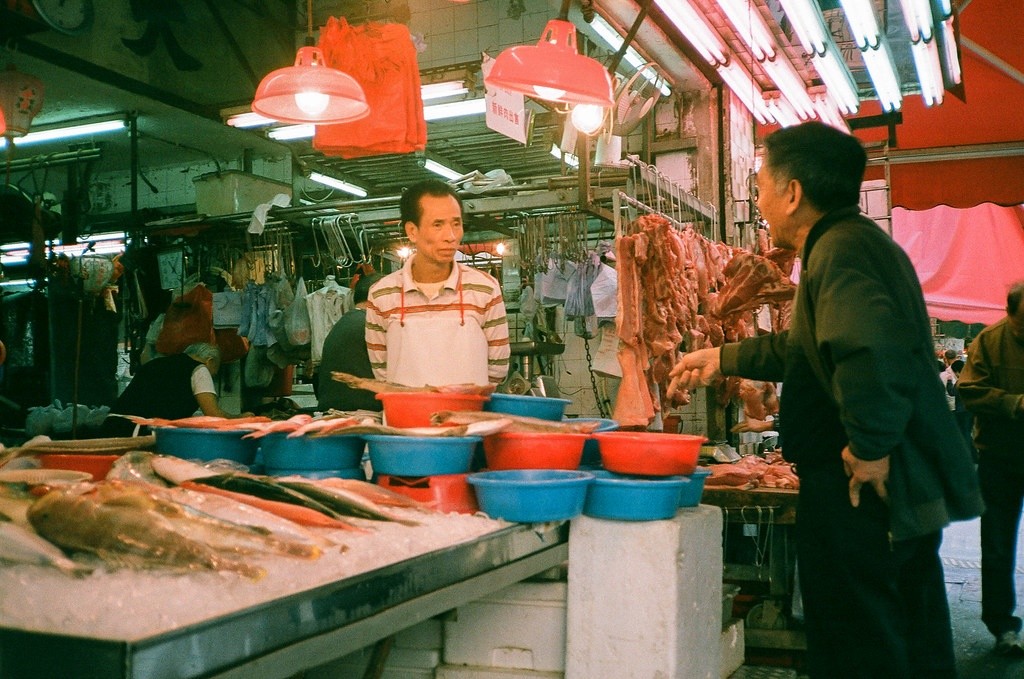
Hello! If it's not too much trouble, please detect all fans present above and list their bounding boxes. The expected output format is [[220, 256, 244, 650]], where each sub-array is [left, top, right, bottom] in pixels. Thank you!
[[605, 61, 664, 137]]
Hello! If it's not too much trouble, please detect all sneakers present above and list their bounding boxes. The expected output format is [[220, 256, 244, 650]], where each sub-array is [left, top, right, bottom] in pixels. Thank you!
[[997, 630, 1024, 654]]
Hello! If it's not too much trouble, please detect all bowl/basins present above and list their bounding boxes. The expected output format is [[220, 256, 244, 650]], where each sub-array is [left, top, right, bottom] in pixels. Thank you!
[[152, 393, 711, 519], [0, 468, 93, 490], [34, 455, 120, 480], [721, 562, 772, 625]]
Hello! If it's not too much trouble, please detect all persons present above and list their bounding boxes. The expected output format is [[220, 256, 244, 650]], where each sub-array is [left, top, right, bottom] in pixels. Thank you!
[[666, 123, 985, 679], [101, 342, 254, 437], [364, 181, 509, 388], [317, 273, 387, 410], [956, 280, 1024, 652], [940, 350, 979, 462]]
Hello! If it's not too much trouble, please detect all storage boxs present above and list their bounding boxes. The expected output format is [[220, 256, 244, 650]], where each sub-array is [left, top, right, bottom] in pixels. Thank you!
[[192, 170, 293, 224], [303, 505, 746, 679], [212, 292, 244, 326]]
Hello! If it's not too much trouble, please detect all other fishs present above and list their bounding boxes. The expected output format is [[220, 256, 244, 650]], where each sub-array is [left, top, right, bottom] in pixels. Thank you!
[[430, 410, 601, 434], [1, 453, 439, 581], [329, 371, 496, 396], [108, 413, 375, 439], [330, 418, 513, 437], [17, 435, 156, 453]]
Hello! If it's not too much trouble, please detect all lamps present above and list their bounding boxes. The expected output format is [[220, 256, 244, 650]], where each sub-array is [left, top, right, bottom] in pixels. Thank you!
[[415, 148, 469, 180], [265, 98, 486, 140], [252, 0, 370, 124], [0, 110, 131, 149], [486, 0, 615, 108], [218, 70, 478, 128], [581, 7, 671, 97], [155, 247, 187, 291], [301, 162, 374, 198], [653, 1, 961, 136], [544, 142, 579, 169]]
